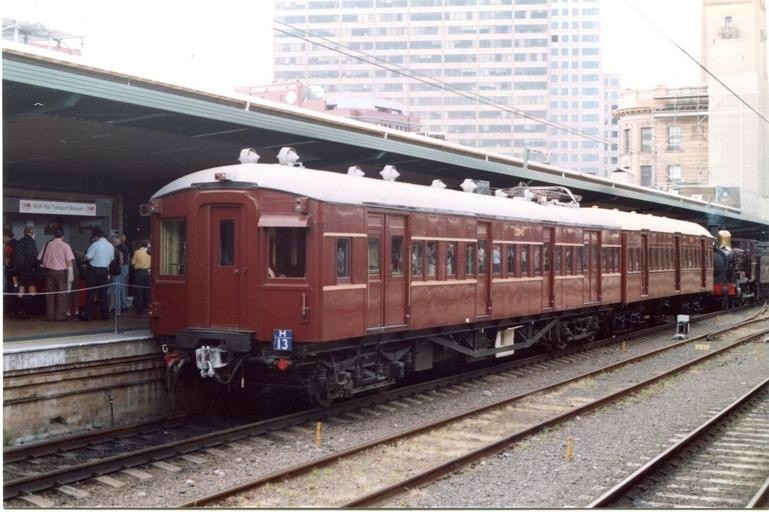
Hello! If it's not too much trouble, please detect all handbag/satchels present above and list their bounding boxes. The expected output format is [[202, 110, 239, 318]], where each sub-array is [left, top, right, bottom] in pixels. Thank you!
[[30, 259, 47, 282]]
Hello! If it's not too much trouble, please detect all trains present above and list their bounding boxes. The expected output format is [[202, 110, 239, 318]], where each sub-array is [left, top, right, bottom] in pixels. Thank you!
[[144, 144, 769, 410]]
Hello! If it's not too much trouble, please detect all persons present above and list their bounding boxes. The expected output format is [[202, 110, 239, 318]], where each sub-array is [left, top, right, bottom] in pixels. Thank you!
[[337, 245, 348, 272], [394, 242, 527, 276], [3, 222, 151, 322]]
